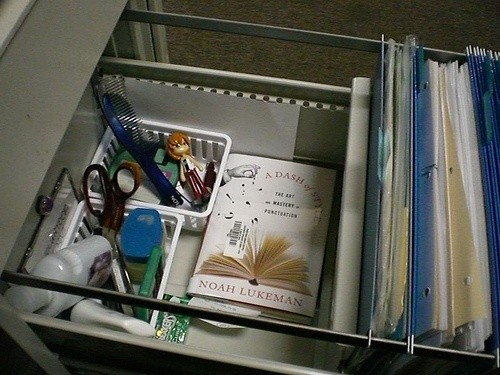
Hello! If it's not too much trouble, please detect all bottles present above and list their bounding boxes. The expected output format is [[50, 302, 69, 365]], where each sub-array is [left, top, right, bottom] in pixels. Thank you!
[[4, 234, 114, 318]]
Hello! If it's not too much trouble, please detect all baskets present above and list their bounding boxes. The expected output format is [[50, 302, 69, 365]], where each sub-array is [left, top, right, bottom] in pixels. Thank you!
[[82, 117, 232, 231], [60, 198, 182, 329]]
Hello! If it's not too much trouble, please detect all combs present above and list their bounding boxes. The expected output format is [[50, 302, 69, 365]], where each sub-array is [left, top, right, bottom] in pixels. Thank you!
[[94, 76, 182, 206]]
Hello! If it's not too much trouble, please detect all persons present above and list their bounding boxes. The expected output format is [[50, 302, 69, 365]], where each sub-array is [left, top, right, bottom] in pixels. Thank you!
[[166, 133, 211, 207], [220, 165, 259, 185]]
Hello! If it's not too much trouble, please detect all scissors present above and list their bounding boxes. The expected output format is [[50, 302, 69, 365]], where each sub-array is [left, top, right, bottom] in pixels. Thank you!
[[82, 160, 142, 317]]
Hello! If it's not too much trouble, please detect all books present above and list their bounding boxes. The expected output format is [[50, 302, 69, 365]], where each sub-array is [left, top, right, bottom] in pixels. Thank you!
[[329, 76, 373, 336], [187, 152, 338, 325]]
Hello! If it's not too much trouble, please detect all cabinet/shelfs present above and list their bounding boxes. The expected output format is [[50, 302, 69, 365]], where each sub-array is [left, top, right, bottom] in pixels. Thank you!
[[0, 0, 500, 375]]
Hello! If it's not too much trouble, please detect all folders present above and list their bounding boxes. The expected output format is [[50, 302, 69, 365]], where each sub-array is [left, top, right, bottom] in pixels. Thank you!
[[340, 33, 417, 375]]
